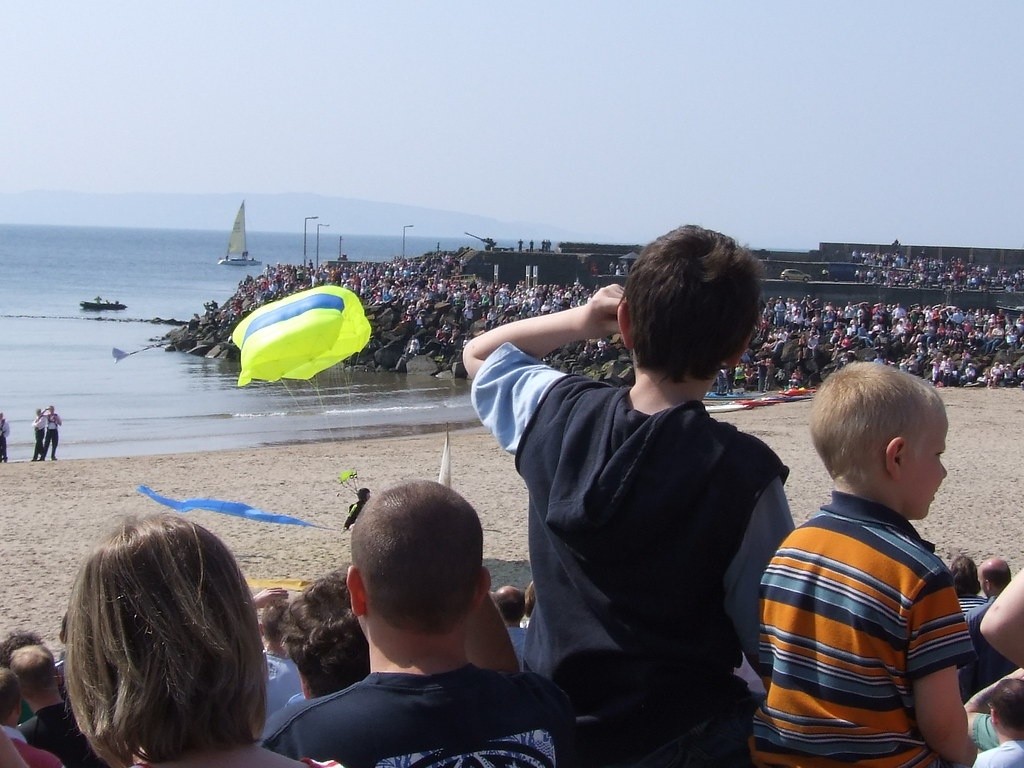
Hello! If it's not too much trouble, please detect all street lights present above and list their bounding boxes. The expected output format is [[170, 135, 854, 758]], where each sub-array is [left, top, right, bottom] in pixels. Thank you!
[[402, 225, 413, 260], [316, 223, 330, 269], [301, 216, 319, 276]]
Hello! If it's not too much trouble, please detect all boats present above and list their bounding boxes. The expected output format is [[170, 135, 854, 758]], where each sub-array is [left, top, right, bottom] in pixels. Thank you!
[[80, 296, 128, 311]]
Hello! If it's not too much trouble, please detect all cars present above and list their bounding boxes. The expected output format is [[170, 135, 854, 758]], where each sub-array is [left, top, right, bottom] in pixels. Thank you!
[[780, 269, 812, 281]]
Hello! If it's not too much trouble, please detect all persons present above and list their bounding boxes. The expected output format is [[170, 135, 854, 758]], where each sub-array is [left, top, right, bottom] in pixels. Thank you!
[[0, 412, 10, 463], [31, 406, 63, 461], [188, 240, 1024, 396], [462, 226, 796, 768], [749, 362, 979, 768], [1, 480, 1024, 768]]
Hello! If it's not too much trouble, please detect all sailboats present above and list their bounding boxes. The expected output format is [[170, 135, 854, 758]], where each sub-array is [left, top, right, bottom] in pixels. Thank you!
[[217, 198, 264, 267]]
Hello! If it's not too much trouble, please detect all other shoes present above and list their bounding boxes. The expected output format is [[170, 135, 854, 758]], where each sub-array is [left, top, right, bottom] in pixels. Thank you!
[[4, 460, 7, 462], [52, 457, 57, 460], [38, 459, 44, 461], [0, 459, 2, 462], [31, 459, 37, 461]]
